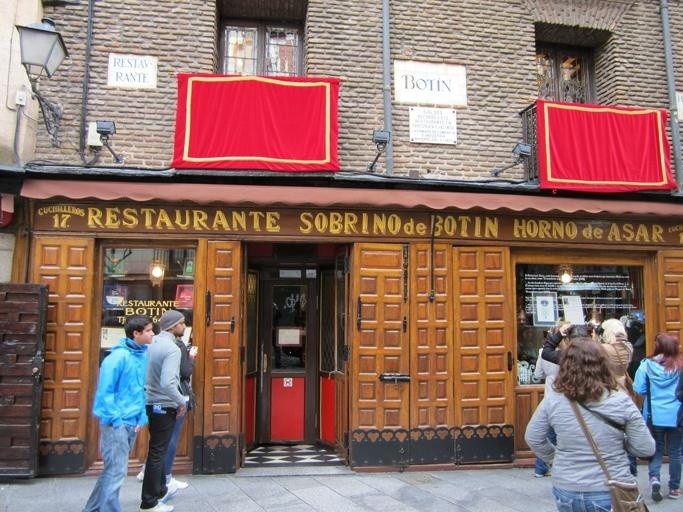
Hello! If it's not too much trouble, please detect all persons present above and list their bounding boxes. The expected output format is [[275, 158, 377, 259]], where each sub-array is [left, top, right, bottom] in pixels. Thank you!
[[523, 318, 683, 512], [140, 310, 187, 512], [137, 335, 198, 489], [517, 310, 645, 363], [83, 315, 155, 512]]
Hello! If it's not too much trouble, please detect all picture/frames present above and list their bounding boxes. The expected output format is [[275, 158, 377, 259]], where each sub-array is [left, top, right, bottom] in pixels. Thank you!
[[102, 285, 129, 308], [175, 284, 194, 308]]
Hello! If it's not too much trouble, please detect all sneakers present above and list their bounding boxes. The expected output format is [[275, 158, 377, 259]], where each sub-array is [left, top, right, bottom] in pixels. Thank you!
[[140, 502, 174, 512], [651, 477, 664, 502], [536, 473, 551, 478], [668, 488, 682, 499], [171, 480, 189, 490], [137, 470, 146, 481], [157, 485, 178, 503]]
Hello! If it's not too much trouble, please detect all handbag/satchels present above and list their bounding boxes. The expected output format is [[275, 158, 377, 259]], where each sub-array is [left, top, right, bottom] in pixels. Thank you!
[[626, 375, 644, 412], [607, 479, 651, 512]]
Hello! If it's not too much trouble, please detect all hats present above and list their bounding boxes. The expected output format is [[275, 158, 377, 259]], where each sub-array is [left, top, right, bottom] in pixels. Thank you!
[[160, 310, 186, 331]]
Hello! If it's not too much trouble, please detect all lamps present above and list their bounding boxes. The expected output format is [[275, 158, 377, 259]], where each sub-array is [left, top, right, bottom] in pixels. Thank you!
[[367, 130, 390, 173], [14, 16, 69, 149], [149, 249, 166, 287], [97, 120, 121, 163], [559, 264, 572, 283], [491, 142, 532, 177]]
